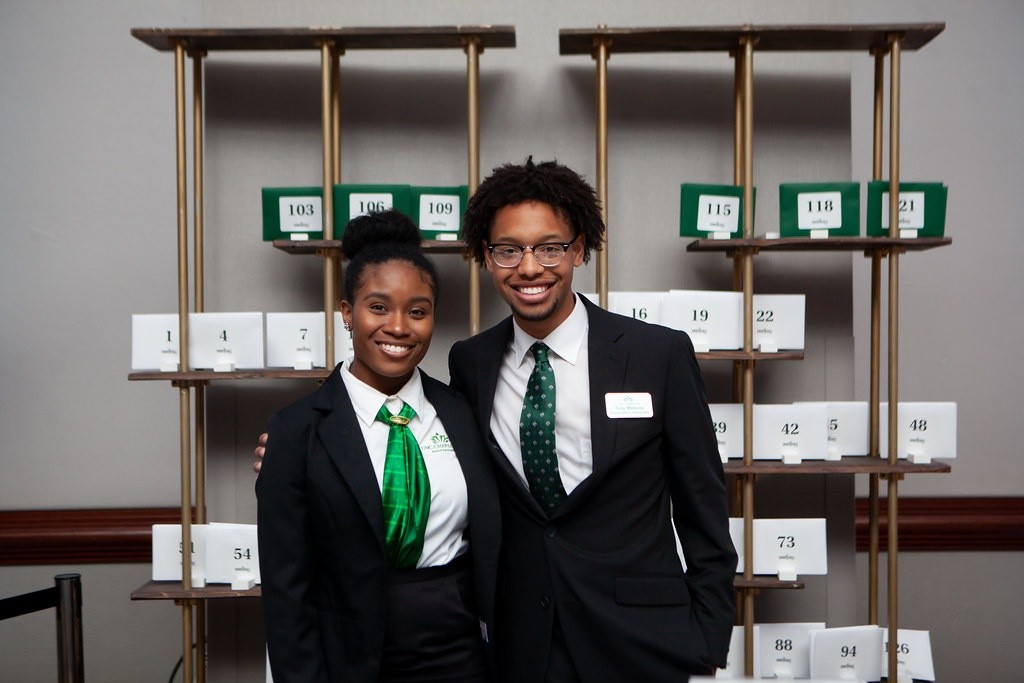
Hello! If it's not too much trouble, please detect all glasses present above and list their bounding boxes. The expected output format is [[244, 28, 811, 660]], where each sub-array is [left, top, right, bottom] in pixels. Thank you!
[[482, 233, 581, 269]]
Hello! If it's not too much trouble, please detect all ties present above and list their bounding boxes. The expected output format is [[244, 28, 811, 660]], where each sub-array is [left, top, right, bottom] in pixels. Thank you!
[[519, 341, 568, 510], [378, 402, 432, 568]]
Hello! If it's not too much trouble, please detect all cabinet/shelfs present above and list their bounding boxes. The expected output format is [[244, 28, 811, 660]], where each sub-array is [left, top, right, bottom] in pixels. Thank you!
[[559, 22, 954, 683], [131, 22, 518, 683]]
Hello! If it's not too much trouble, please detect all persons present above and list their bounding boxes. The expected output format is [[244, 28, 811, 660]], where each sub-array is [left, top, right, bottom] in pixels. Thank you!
[[252, 154, 740, 683], [254, 207, 502, 683]]
